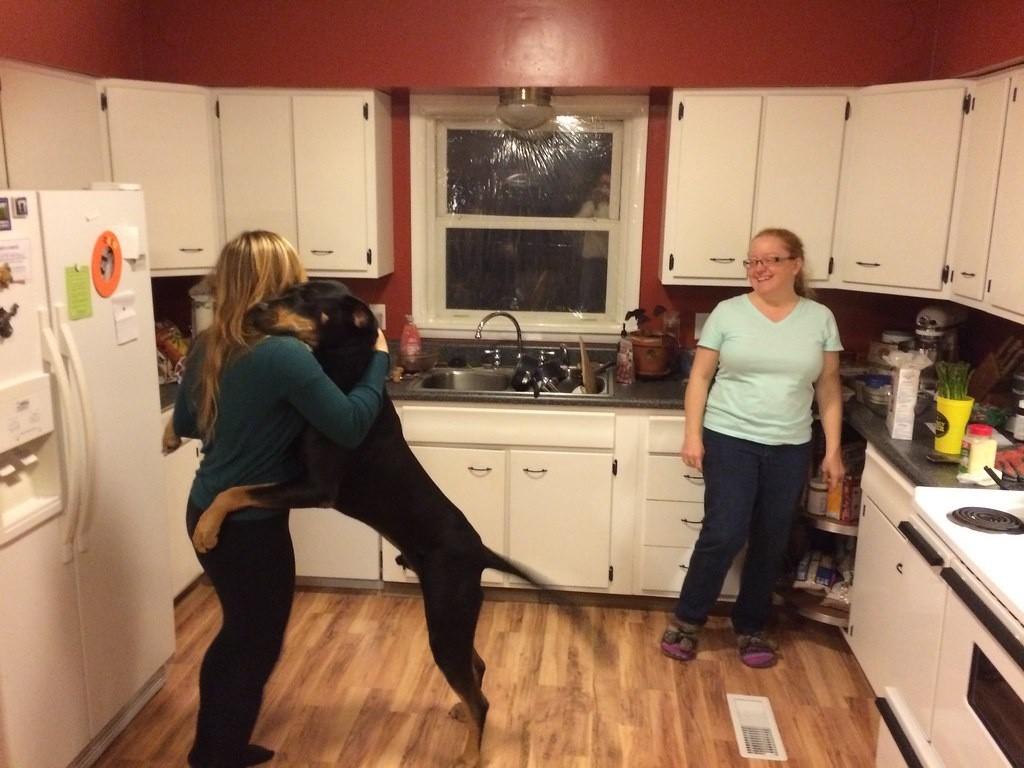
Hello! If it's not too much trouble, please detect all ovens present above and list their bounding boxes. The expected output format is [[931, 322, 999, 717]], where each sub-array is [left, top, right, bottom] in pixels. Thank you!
[[930, 557, 1024, 768]]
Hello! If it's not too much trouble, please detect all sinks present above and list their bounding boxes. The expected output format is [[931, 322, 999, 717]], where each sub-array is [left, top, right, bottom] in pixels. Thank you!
[[410, 366, 609, 400]]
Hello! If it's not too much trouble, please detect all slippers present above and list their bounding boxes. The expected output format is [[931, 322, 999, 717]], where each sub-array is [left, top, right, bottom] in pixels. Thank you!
[[661, 614, 703, 661], [735, 633, 775, 668]]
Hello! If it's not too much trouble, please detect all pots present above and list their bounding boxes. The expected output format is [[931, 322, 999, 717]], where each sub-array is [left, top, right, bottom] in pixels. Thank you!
[[535, 360, 561, 393], [512, 354, 540, 398]]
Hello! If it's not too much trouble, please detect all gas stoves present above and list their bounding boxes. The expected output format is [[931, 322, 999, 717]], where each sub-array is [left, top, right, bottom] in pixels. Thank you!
[[912, 486, 1024, 626]]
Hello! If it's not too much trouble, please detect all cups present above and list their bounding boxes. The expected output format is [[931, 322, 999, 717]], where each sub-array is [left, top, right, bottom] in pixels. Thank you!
[[934, 395, 975, 454]]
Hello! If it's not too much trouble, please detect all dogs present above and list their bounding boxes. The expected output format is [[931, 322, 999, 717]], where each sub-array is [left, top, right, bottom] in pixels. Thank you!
[[160, 279, 616, 768]]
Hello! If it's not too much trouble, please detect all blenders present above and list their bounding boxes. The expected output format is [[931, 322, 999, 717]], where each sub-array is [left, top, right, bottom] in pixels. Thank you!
[[915, 300, 972, 395]]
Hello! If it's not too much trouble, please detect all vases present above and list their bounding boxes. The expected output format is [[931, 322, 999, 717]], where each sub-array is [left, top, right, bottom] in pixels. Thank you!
[[627, 330, 678, 380]]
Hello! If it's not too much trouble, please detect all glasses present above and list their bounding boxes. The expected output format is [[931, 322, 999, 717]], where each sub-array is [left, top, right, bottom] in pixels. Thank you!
[[743, 256, 796, 269]]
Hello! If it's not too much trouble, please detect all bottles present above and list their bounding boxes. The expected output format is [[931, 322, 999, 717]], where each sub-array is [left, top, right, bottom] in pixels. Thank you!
[[1013, 400, 1024, 444], [807, 477, 829, 517], [958, 424, 997, 474]]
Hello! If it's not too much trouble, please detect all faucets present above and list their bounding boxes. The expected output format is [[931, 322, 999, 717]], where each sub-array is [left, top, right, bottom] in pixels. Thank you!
[[474, 310, 525, 370]]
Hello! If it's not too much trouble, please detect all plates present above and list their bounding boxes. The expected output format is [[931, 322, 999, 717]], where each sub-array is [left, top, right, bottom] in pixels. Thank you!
[[841, 382, 854, 403]]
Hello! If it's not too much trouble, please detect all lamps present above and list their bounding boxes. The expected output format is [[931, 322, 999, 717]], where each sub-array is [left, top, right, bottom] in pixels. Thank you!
[[495, 88, 557, 132]]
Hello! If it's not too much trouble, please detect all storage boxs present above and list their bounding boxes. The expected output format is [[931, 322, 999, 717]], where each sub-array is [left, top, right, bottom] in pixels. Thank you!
[[886, 367, 920, 440]]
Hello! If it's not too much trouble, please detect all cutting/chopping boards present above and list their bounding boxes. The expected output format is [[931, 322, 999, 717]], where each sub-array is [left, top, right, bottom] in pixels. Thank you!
[[579, 334, 597, 394]]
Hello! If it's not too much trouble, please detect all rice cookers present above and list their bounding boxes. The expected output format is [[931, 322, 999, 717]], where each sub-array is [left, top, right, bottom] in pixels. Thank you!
[[624, 330, 677, 379]]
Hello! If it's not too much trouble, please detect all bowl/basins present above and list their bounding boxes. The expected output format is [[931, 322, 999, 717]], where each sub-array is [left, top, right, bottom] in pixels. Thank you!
[[861, 395, 887, 418], [389, 348, 441, 373]]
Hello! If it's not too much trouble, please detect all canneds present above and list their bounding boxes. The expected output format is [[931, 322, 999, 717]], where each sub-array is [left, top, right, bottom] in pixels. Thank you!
[[805, 478, 828, 516], [958, 424, 997, 474]]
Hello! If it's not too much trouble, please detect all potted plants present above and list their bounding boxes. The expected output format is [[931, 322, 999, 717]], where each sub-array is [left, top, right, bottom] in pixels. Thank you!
[[933, 359, 974, 456]]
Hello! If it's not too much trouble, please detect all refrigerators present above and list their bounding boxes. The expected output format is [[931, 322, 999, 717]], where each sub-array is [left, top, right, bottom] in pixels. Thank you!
[[0, 182, 175, 768]]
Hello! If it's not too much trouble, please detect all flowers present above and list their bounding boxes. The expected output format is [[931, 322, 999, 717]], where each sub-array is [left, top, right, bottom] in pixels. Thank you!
[[626, 304, 666, 337]]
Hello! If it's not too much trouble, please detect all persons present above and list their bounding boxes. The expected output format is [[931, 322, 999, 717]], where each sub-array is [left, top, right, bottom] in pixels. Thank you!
[[171, 229, 390, 768], [662, 228, 846, 670], [573, 170, 611, 313]]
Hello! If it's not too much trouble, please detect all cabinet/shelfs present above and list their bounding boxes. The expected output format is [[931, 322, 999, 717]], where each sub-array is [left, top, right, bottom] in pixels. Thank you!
[[166, 406, 1024, 768], [0, 56, 393, 278], [657, 63, 1024, 325]]
